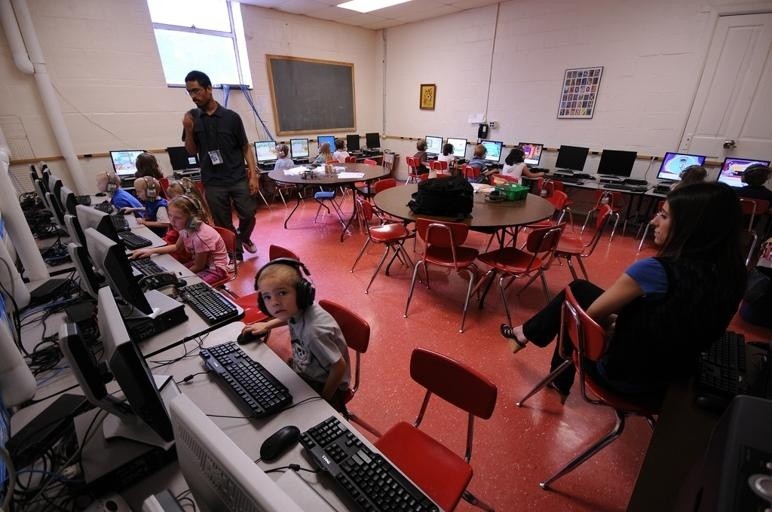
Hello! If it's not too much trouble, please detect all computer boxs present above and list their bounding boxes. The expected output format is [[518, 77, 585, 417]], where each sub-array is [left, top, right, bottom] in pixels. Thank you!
[[62, 289, 185, 346], [71, 376, 181, 496], [174, 171, 201, 180]]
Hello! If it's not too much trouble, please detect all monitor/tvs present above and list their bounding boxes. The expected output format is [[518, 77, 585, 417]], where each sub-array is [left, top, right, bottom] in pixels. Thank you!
[[109, 150, 147, 175], [59, 285, 184, 450], [252, 132, 381, 170], [29, 160, 155, 316], [167, 147, 199, 172], [171, 392, 300, 512]]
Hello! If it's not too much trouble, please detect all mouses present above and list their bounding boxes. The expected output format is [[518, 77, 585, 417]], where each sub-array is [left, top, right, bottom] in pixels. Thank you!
[[698, 393, 726, 413], [237, 331, 266, 345], [174, 279, 187, 288], [258, 425, 299, 462]]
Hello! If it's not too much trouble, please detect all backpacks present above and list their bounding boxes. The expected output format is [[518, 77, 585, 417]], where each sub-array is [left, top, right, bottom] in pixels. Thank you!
[[407, 175, 474, 221]]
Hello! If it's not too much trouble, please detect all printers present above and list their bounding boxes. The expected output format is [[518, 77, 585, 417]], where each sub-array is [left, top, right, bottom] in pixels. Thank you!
[[688, 394, 771, 512]]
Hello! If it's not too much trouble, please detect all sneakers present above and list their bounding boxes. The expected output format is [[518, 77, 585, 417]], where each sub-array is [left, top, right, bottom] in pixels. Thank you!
[[226, 254, 243, 273], [237, 228, 257, 253]]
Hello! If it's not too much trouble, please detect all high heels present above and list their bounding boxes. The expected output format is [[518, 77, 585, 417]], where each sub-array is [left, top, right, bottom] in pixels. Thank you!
[[500, 322, 526, 354], [546, 381, 571, 406]]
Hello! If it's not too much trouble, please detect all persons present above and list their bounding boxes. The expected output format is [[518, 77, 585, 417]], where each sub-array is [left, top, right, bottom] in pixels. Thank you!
[[464, 143, 501, 185], [670, 163, 710, 191], [269, 133, 357, 179], [90, 147, 235, 297], [430, 142, 459, 179], [176, 68, 262, 275], [239, 256, 356, 403], [733, 159, 772, 230], [411, 137, 435, 177], [497, 148, 548, 188], [498, 175, 750, 408]]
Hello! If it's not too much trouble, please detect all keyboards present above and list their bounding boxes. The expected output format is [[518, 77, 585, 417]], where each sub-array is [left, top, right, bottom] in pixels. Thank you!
[[177, 280, 239, 328], [295, 413, 439, 512], [693, 332, 752, 395], [200, 341, 289, 426], [100, 200, 177, 289]]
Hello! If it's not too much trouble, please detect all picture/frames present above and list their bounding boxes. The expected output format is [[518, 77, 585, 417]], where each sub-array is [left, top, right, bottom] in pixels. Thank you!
[[418, 83, 436, 110], [556, 66, 604, 118]]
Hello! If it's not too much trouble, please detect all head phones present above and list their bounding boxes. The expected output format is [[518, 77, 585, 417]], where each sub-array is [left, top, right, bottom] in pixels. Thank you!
[[166, 194, 203, 228], [301, 170, 314, 179], [252, 257, 315, 315]]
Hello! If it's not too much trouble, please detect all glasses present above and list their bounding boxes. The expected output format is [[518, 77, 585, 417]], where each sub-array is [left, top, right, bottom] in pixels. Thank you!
[[186, 87, 204, 95]]
